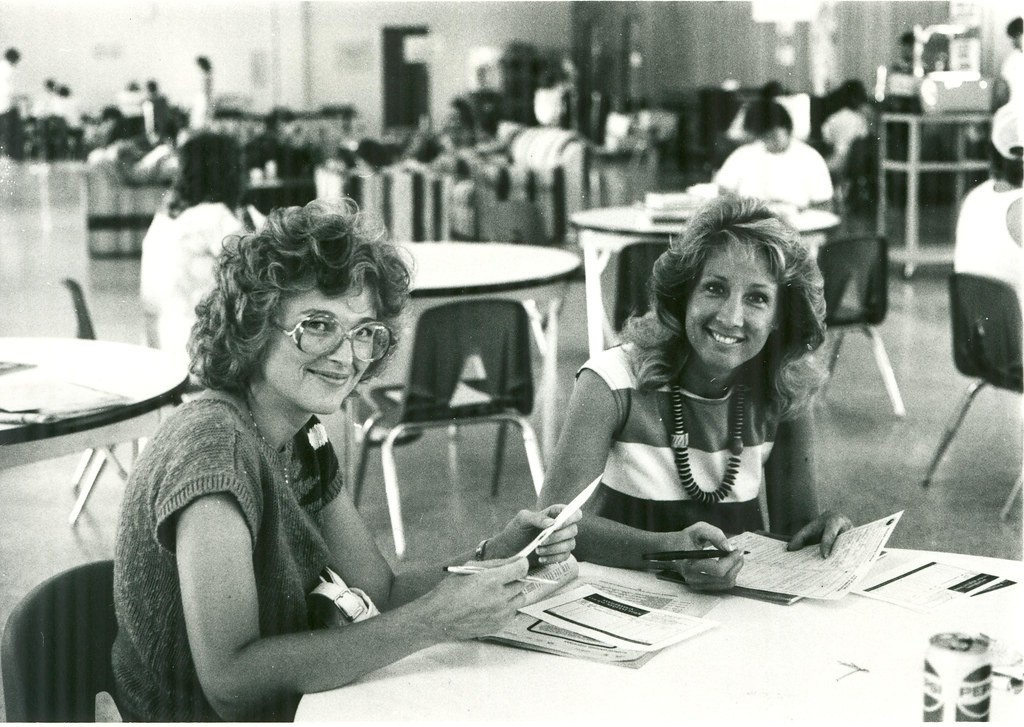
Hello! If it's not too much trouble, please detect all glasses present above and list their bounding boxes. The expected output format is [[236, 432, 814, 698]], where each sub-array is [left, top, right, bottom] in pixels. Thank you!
[[268, 311, 397, 363]]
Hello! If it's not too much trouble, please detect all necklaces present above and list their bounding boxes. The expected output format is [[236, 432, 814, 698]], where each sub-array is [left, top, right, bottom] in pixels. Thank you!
[[245, 398, 290, 489], [667, 373, 746, 506]]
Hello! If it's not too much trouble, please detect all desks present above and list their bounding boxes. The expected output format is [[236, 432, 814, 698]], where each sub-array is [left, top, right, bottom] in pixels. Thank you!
[[292, 548, 1024, 727], [0, 336, 188, 470], [567, 205, 843, 363], [393, 240, 583, 466]]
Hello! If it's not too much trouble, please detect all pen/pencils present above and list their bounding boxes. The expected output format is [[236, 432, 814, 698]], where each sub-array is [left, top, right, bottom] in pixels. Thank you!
[[442, 565, 557, 586], [642, 546, 751, 562]]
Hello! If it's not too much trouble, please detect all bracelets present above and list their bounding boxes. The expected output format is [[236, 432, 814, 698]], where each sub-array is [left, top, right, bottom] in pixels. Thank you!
[[476, 538, 486, 562]]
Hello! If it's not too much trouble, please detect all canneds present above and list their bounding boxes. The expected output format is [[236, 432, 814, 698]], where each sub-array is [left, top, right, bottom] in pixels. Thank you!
[[922, 630, 993, 722]]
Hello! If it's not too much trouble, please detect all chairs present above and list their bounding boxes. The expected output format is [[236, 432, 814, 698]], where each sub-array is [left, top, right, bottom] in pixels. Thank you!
[[0, 89, 1024, 727]]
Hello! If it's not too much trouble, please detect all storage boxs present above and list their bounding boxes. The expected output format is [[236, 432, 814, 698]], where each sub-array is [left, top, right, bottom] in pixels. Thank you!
[[917, 77, 996, 115]]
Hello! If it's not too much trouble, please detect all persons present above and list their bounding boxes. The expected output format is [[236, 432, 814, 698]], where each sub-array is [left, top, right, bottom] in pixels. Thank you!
[[111, 194, 583, 723], [0, 47, 221, 158], [714, 18, 1024, 324], [392, 57, 581, 158], [138, 132, 254, 368], [536, 195, 855, 592]]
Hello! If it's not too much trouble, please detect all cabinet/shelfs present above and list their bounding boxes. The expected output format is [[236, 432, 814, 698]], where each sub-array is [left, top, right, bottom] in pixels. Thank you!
[[872, 113, 994, 281]]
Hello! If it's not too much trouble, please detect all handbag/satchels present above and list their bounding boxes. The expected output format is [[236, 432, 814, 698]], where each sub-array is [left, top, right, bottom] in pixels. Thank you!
[[307, 564, 382, 629]]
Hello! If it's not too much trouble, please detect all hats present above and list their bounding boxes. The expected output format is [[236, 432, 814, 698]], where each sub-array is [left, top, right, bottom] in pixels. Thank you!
[[989, 98, 1024, 161]]
